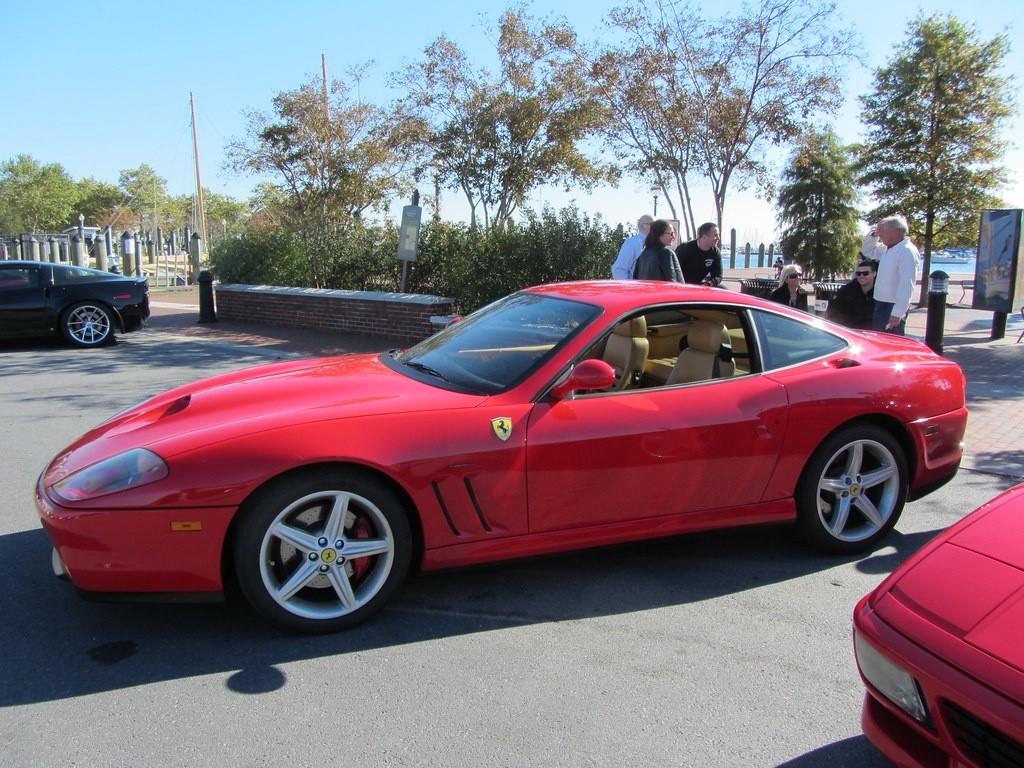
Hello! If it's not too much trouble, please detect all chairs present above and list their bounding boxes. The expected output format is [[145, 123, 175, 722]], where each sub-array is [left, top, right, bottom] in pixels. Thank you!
[[28, 269, 37, 284], [667, 320, 736, 386], [585, 315, 649, 394]]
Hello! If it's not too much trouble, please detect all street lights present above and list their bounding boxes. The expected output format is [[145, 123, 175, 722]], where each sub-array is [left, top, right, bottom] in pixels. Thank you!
[[79, 213, 85, 254], [221, 219, 227, 252], [649, 178, 662, 220]]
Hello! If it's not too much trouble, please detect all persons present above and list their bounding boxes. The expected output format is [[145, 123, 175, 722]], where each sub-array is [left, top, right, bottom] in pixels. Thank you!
[[825, 261, 879, 330], [610, 215, 655, 280], [772, 257, 784, 281], [632, 219, 686, 285], [860, 214, 920, 338], [675, 222, 730, 291], [768, 264, 809, 315]]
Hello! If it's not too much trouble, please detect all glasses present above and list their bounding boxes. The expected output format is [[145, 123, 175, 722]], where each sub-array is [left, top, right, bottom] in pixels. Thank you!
[[855, 271, 873, 276], [787, 273, 803, 278], [664, 232, 676, 236]]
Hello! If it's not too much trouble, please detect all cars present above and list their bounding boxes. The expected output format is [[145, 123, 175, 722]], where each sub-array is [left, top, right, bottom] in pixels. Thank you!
[[853, 479, 1024, 768], [0, 258, 150, 348]]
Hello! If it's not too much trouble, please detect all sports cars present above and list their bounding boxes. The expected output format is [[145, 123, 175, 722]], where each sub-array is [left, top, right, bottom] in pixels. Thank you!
[[34, 280, 967, 636]]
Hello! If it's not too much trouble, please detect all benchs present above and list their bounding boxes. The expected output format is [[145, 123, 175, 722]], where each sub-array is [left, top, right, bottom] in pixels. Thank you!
[[645, 333, 750, 383]]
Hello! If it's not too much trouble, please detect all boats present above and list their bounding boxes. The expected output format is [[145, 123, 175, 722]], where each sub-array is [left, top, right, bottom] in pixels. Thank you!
[[932, 254, 968, 263], [721, 248, 730, 259]]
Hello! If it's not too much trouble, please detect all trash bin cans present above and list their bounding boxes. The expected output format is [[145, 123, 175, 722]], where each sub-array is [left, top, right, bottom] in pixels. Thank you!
[[737, 279, 780, 299], [812, 283, 847, 317]]
